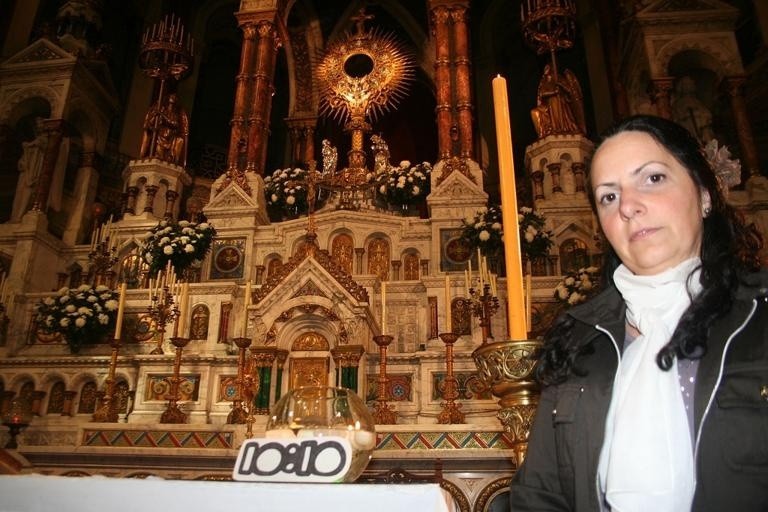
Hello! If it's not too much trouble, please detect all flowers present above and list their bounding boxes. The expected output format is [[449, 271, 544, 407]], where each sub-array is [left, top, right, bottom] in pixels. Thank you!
[[34, 281, 126, 335], [142, 217, 216, 261], [462, 197, 557, 249], [263, 167, 327, 207], [553, 263, 603, 307], [368, 158, 436, 195]]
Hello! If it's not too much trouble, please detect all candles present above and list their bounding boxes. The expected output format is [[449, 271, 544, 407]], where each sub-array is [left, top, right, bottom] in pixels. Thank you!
[[88, 213, 124, 260], [443, 272, 453, 333], [493, 72, 532, 341], [148, 259, 185, 310], [175, 281, 190, 338], [378, 279, 389, 333], [114, 283, 127, 338], [241, 281, 252, 338], [463, 246, 498, 299]]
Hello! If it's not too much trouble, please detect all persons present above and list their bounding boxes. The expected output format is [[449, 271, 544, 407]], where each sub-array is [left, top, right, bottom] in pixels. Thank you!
[[506, 112, 768, 512]]
